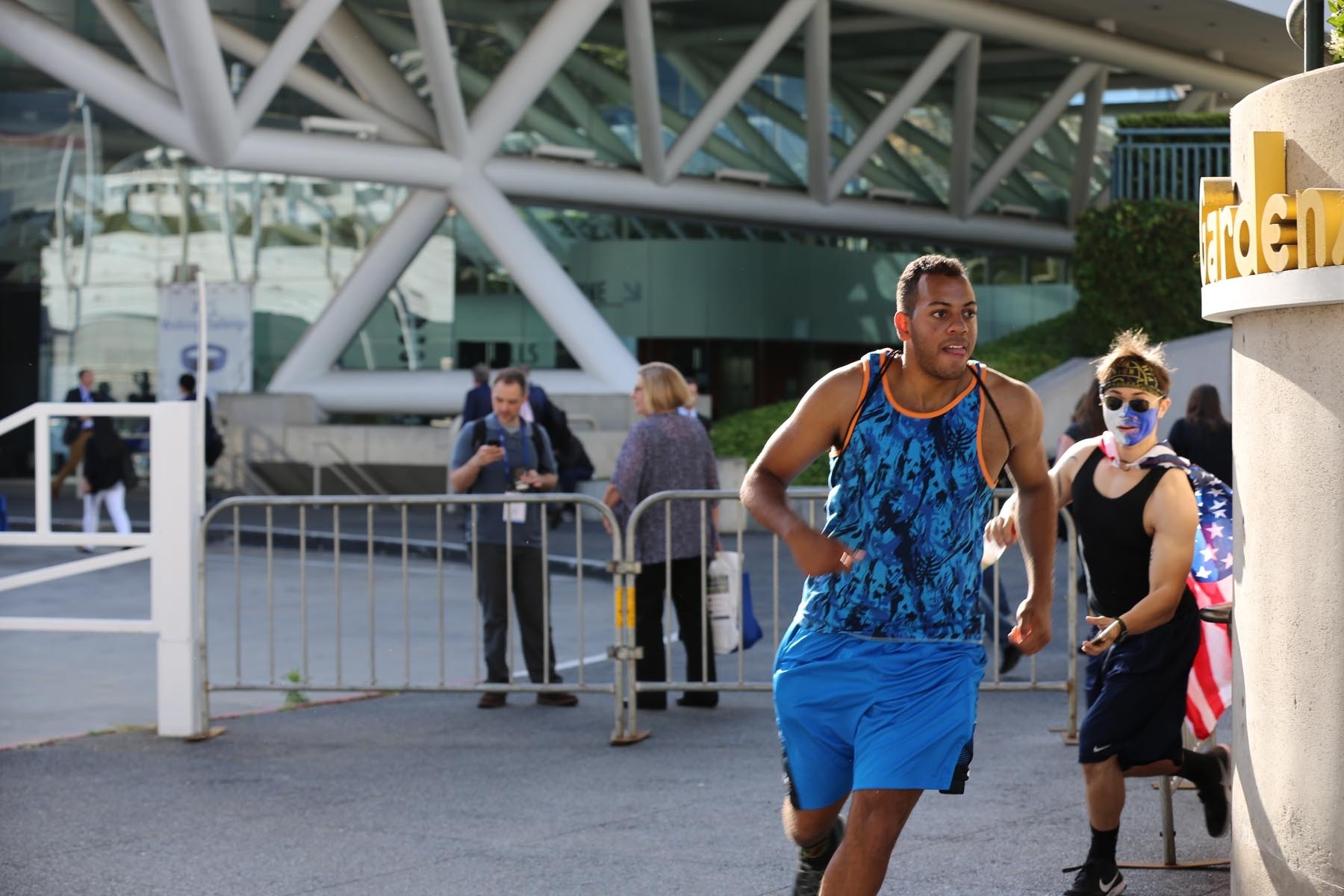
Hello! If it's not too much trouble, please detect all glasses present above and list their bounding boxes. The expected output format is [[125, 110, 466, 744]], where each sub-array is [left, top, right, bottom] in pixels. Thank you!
[[1101, 393, 1166, 414]]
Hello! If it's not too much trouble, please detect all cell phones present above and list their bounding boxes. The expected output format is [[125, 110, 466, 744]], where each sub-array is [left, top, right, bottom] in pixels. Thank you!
[[487, 435, 503, 446], [1080, 620, 1119, 649]]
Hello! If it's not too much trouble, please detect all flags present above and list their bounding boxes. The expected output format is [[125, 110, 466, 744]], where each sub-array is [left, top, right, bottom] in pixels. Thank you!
[[1098, 424, 1233, 740]]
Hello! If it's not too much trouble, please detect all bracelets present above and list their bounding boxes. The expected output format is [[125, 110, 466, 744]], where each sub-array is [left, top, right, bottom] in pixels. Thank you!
[[1112, 617, 1129, 645]]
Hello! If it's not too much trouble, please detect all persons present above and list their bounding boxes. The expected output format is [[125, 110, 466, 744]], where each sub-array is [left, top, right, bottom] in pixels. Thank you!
[[179, 375, 212, 472], [52, 370, 156, 552], [984, 328, 1233, 896], [446, 363, 597, 708], [737, 252, 1059, 896], [676, 378, 711, 437], [603, 362, 726, 712]]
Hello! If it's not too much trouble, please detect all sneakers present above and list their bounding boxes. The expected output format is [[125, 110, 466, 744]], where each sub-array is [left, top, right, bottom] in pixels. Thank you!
[[793, 812, 848, 896], [1197, 742, 1232, 837], [1061, 859, 1127, 896]]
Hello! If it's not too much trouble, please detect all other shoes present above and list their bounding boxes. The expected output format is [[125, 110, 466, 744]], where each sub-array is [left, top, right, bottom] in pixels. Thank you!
[[561, 510, 574, 523], [675, 696, 717, 709], [51, 482, 59, 499], [73, 545, 93, 554], [537, 691, 578, 706], [120, 546, 133, 551], [621, 697, 668, 710], [478, 692, 507, 707], [998, 644, 1021, 674]]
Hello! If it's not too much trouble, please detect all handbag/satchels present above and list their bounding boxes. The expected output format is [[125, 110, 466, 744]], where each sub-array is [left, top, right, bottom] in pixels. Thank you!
[[206, 424, 225, 467], [707, 551, 744, 655], [727, 573, 763, 654], [118, 454, 138, 491]]
